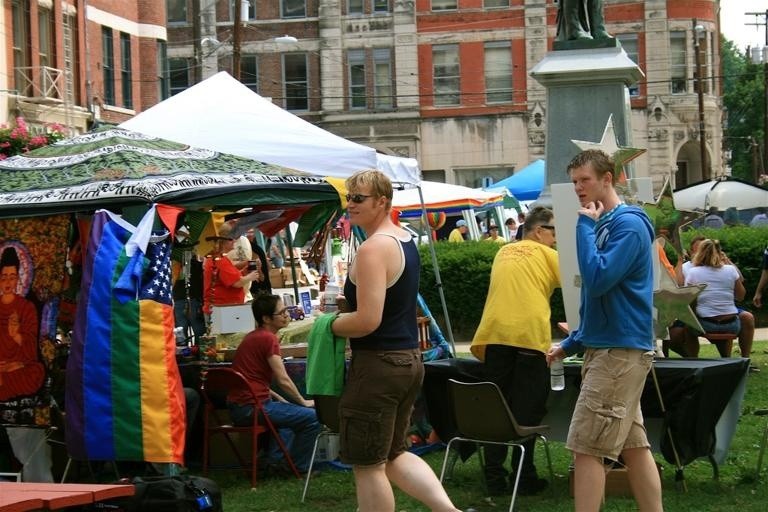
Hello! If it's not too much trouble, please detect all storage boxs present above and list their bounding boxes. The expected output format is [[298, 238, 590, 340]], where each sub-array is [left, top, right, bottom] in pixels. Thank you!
[[311, 428, 343, 464]]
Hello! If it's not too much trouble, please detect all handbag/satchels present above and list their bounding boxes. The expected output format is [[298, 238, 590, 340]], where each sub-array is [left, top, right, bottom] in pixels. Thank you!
[[121, 474, 222, 510]]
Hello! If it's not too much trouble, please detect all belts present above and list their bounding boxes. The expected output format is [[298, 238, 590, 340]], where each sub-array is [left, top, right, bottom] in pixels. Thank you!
[[704, 317, 738, 324]]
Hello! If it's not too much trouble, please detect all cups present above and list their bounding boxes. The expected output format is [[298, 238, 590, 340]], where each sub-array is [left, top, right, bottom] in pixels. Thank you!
[[199, 336, 217, 362], [247, 261, 256, 275]]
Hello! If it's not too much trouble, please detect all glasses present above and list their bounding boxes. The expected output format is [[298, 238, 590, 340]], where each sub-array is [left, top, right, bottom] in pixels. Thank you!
[[271, 307, 287, 316], [346, 194, 374, 203]]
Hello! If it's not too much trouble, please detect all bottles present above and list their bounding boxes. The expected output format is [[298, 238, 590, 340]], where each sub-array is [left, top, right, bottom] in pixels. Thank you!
[[324, 276, 339, 313], [550, 345, 566, 392]]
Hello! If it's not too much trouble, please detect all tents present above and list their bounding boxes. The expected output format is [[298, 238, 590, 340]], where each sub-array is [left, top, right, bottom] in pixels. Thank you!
[[486, 159, 547, 238], [391, 179, 508, 241]]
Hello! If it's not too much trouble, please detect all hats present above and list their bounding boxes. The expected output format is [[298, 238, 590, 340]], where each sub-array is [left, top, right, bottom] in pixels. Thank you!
[[457, 220, 469, 226]]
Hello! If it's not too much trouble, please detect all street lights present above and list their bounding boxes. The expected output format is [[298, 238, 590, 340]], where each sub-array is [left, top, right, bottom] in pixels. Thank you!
[[693, 24, 709, 182]]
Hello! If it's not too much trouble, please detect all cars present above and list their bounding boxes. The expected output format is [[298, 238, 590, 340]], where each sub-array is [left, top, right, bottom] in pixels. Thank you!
[[398, 221, 430, 248]]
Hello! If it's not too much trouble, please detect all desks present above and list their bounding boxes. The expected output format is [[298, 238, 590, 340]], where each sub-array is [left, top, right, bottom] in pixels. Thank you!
[[414, 351, 752, 496], [0, 474, 140, 511], [204, 342, 313, 470], [279, 315, 319, 346]]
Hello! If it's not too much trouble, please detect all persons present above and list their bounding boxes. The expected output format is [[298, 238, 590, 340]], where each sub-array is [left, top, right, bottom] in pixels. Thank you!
[[471, 207, 560, 495], [173, 218, 324, 512], [0, 247, 45, 403], [313, 167, 462, 512], [753, 245, 767, 308], [544, 148, 663, 512], [448, 211, 526, 243], [690, 207, 767, 228], [657, 231, 759, 372]]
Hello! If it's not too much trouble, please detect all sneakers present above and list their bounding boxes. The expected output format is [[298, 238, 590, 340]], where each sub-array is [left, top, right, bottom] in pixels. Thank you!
[[513, 479, 548, 494], [485, 486, 505, 495]]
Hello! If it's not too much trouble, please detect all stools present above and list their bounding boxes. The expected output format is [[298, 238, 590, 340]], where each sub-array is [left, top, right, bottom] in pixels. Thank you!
[[697, 330, 738, 360]]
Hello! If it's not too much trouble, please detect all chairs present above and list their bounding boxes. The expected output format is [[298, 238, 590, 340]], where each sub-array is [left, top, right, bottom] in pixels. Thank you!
[[292, 357, 345, 504], [193, 362, 304, 496], [433, 373, 558, 511]]
